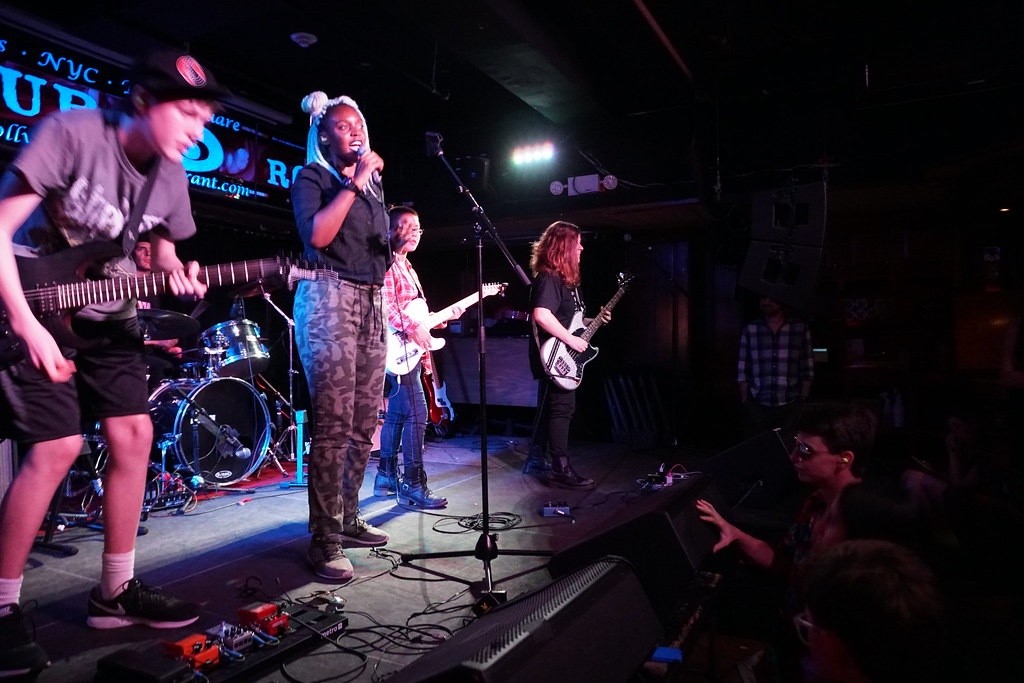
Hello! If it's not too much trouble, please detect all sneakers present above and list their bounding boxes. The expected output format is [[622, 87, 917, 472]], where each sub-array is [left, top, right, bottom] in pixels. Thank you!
[[308, 543, 354, 579], [87, 577, 200, 628], [0, 599, 51, 675], [342, 520, 389, 544]]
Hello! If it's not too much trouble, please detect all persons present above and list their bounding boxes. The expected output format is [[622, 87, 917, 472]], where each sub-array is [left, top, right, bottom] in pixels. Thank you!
[[521, 222, 611, 491], [0, 49, 230, 679], [373, 206, 467, 509], [293, 92, 395, 581], [695, 273, 1024, 683]]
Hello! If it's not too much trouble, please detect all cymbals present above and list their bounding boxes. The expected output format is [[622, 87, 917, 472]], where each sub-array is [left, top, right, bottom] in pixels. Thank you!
[[229, 276, 284, 299], [136, 309, 201, 340]]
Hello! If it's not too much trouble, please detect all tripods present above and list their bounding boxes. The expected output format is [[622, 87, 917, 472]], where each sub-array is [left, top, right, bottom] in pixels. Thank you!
[[138, 386, 256, 522], [400, 133, 557, 591], [260, 284, 298, 466]]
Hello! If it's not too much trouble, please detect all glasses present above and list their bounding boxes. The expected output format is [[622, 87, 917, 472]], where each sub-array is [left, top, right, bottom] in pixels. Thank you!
[[791, 437, 837, 460], [795, 612, 830, 646], [412, 228, 423, 236]]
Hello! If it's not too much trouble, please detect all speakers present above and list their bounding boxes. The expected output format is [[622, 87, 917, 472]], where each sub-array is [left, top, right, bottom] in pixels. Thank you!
[[0, 437, 20, 504], [370, 397, 390, 457], [381, 426, 799, 683]]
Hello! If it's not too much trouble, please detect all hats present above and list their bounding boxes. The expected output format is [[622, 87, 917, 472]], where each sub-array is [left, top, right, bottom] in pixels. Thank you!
[[128, 50, 233, 102]]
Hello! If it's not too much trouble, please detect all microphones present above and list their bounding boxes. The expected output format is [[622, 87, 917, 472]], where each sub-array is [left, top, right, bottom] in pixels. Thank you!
[[235, 447, 252, 459], [231, 295, 239, 318], [357, 147, 382, 190]]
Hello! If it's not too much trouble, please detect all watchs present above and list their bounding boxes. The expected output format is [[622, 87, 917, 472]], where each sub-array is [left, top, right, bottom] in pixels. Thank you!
[[341, 176, 361, 197]]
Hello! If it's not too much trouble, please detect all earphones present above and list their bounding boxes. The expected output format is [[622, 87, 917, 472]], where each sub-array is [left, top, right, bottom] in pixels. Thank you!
[[320, 136, 328, 142], [838, 458, 849, 468]]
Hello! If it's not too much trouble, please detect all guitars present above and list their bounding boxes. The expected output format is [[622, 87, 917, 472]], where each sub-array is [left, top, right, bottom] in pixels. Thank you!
[[540, 272, 632, 391], [385, 282, 509, 376], [0, 249, 339, 371]]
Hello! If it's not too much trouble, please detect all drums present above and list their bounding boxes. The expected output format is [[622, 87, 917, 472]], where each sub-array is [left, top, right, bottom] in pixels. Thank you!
[[147, 377, 271, 486], [198, 318, 271, 380], [177, 362, 207, 379]]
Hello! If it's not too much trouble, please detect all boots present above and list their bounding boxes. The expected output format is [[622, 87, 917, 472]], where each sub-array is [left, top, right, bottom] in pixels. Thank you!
[[374, 456, 405, 496], [399, 466, 448, 507], [548, 454, 594, 490], [523, 443, 549, 487]]
